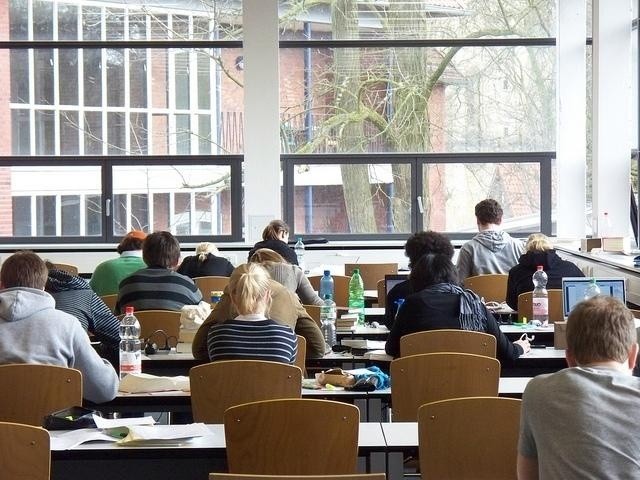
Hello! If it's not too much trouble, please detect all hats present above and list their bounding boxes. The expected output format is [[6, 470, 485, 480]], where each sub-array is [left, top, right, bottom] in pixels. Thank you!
[[126, 231, 147, 241]]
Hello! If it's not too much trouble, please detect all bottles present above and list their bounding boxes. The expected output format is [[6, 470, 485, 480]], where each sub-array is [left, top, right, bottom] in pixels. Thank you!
[[319, 293, 335, 349], [117, 307, 143, 384], [294, 238, 305, 275], [348, 270, 365, 324], [583, 279, 601, 302], [320, 270, 336, 306], [533, 266, 548, 330]]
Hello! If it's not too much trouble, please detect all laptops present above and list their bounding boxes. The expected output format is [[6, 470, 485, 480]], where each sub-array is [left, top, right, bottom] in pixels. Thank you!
[[562, 277, 626, 321], [385, 275, 408, 299]]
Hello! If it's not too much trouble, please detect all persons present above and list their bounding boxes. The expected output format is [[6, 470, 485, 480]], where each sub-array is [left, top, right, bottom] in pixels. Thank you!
[[191, 218, 329, 405], [89, 219, 236, 313], [384, 230, 463, 331], [1, 251, 128, 404], [456, 198, 526, 285], [385, 254, 530, 363], [506, 231, 586, 322], [517, 295, 640, 480]]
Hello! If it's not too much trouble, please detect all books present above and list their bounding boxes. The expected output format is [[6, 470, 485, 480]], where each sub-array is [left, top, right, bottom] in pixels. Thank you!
[[341, 340, 386, 351], [335, 312, 359, 336]]
